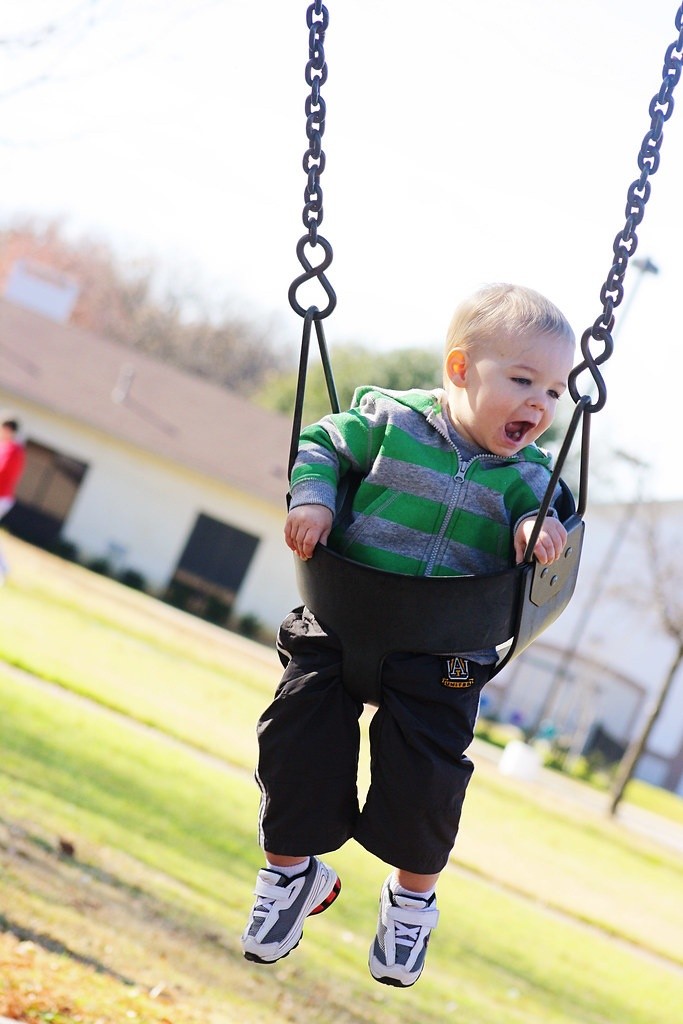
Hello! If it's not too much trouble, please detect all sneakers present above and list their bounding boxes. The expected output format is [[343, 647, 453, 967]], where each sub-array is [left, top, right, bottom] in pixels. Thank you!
[[369, 873, 440, 987], [240, 855, 341, 964]]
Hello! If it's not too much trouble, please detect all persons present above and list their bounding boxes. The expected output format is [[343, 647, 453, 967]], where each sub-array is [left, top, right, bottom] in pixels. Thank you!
[[0, 420, 24, 576], [242, 284, 578, 985]]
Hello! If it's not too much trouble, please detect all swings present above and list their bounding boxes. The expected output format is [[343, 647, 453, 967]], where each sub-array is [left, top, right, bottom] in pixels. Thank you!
[[287, 0, 683, 683]]
